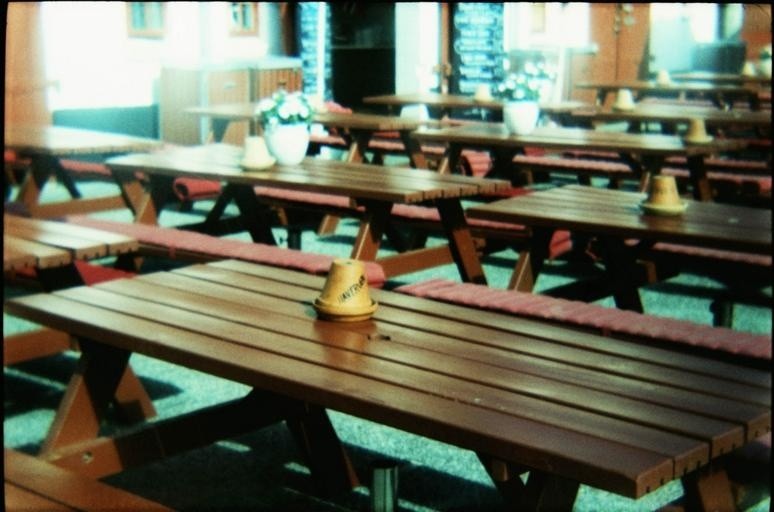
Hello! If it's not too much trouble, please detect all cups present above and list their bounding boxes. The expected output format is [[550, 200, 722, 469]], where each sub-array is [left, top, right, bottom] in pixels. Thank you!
[[241, 135, 273, 168], [685, 117, 709, 142], [315, 258, 374, 311], [614, 86, 635, 109], [643, 173, 683, 210]]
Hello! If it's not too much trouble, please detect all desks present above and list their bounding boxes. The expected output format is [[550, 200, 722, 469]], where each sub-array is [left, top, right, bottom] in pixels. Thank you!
[[1, 71, 773, 512]]
